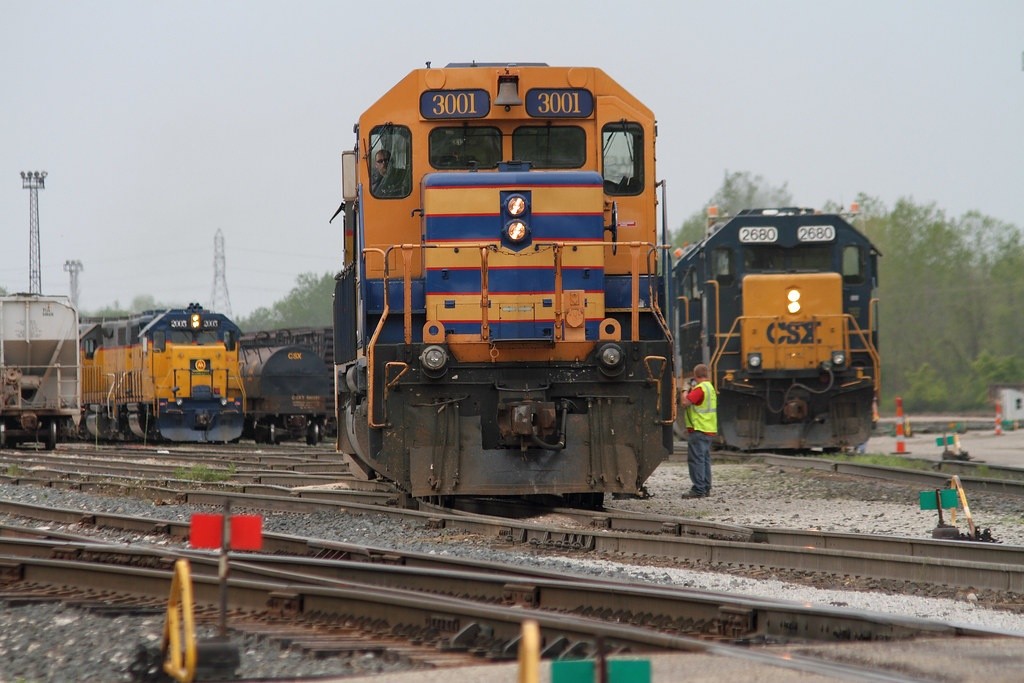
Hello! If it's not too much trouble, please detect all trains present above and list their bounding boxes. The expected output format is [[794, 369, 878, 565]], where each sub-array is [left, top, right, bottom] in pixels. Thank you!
[[675, 202, 887, 454], [78, 301, 247, 439], [0, 292, 81, 450], [329, 55, 678, 515]]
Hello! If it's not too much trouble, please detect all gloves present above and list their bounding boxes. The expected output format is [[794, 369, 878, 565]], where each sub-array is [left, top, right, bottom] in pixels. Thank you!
[[681, 381, 691, 392]]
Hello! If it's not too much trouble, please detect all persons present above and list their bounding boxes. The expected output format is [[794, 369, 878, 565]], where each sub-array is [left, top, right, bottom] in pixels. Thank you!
[[371, 150, 407, 197], [679, 364, 717, 498]]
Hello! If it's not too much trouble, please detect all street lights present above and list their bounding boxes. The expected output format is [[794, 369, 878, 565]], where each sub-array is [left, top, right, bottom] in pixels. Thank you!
[[20, 168, 48, 294], [63, 259, 83, 303]]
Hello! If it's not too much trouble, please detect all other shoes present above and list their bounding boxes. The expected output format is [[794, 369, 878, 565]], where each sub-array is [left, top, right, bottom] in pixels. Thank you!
[[682, 490, 710, 499]]
